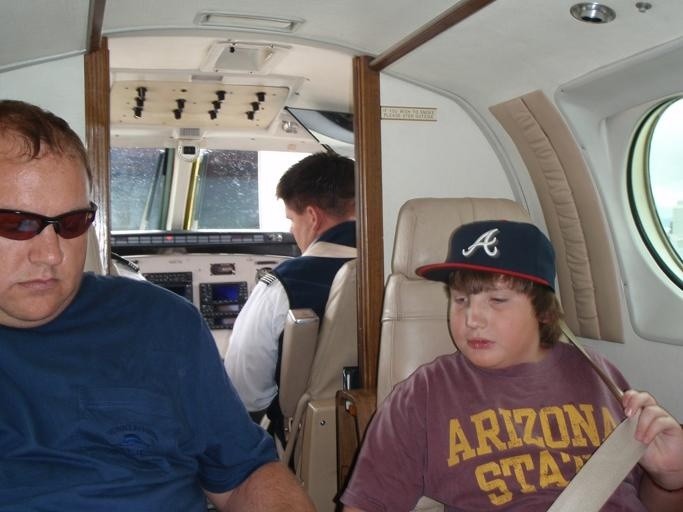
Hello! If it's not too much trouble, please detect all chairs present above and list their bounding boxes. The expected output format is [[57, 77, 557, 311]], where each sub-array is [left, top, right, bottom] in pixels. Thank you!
[[271, 256, 360, 512], [374, 195, 539, 415]]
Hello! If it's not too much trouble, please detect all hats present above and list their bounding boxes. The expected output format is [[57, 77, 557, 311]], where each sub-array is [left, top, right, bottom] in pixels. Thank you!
[[415, 220, 555, 291]]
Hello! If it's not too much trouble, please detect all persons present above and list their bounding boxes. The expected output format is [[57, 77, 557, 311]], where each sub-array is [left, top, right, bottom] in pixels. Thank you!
[[340, 219, 682, 511], [0, 97, 320, 511], [221, 149, 355, 471]]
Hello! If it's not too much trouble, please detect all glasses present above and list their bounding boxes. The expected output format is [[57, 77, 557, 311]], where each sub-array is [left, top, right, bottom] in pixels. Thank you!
[[1, 201, 97, 240]]
